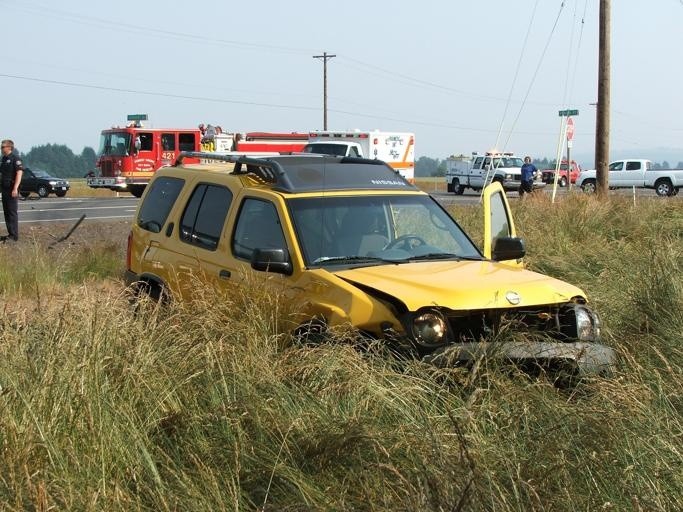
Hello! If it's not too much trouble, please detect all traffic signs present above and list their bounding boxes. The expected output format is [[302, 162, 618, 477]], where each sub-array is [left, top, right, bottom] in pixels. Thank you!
[[556, 109, 579, 117], [125, 113, 148, 121]]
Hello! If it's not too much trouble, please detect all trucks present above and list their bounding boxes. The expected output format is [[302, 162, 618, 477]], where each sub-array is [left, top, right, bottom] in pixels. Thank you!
[[300, 130, 416, 187]]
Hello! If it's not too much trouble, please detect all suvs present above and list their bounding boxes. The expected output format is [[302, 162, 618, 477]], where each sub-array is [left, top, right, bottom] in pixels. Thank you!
[[19, 165, 71, 200], [539, 160, 581, 187], [123, 147, 609, 357], [440, 149, 546, 196]]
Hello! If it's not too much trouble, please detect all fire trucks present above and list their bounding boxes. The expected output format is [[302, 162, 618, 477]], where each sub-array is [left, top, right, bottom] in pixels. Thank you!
[[82, 122, 314, 201]]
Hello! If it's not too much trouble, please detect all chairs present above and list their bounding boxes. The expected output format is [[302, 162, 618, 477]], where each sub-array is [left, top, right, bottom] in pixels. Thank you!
[[335, 205, 391, 257]]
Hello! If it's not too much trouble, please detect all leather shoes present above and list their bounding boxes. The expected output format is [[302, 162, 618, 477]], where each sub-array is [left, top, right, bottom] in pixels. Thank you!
[[4, 235, 10, 240], [4, 239, 16, 242]]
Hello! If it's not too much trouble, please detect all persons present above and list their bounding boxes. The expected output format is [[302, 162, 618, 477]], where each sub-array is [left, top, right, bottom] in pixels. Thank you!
[[196, 124, 241, 150], [0, 139, 24, 246], [518, 156, 540, 202]]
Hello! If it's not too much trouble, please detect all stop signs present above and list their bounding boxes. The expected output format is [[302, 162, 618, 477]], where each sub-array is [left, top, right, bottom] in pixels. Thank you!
[[566, 116, 574, 140]]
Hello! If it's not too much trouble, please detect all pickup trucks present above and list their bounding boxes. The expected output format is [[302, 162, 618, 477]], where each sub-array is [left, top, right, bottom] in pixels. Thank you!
[[574, 156, 682, 197]]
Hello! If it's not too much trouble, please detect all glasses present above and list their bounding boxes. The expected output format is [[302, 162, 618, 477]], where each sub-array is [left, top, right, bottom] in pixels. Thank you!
[[2, 146, 8, 148]]
